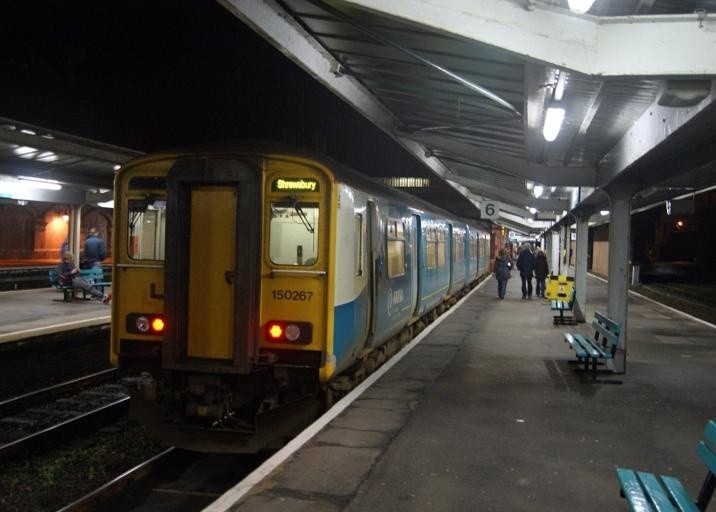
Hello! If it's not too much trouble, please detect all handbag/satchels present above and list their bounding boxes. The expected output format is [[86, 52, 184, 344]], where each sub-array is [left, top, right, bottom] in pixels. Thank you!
[[506, 261, 512, 270], [55, 274, 71, 286]]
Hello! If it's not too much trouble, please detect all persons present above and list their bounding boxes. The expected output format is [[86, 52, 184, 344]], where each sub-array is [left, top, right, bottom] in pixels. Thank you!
[[83, 227, 106, 299], [59, 252, 112, 304], [491, 248, 513, 300], [503, 242, 524, 270], [515, 244, 535, 302], [533, 247, 549, 299]]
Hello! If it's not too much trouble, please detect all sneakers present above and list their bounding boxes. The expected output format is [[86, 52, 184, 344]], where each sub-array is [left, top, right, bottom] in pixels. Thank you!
[[102, 292, 112, 304], [522, 294, 544, 299]]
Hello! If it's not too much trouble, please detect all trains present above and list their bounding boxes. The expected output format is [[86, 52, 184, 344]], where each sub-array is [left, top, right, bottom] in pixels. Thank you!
[[109, 143, 490, 453]]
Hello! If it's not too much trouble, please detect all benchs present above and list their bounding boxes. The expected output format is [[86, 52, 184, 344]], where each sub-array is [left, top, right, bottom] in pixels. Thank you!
[[615, 419, 716, 512], [48, 266, 112, 302], [550, 287, 627, 387]]
